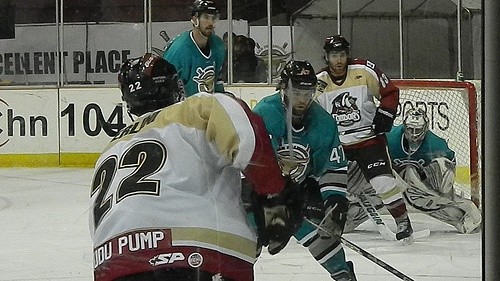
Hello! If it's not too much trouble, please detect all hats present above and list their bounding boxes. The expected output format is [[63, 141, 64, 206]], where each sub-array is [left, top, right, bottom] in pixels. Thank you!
[[234, 34, 248, 46]]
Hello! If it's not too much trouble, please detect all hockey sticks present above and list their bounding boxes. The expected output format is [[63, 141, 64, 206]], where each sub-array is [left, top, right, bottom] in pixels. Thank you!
[[93, 108, 119, 136], [301, 215, 417, 280], [357, 193, 430, 243]]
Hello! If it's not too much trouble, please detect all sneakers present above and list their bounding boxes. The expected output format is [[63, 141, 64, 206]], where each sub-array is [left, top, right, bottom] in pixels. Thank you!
[[395, 213, 414, 247]]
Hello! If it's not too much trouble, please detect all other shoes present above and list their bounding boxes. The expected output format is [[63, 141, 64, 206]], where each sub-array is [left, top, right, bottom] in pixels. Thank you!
[[330, 260, 357, 281]]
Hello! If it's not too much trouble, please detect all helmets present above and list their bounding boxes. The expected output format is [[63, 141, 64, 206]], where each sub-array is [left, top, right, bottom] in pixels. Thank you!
[[280, 59, 317, 90], [404, 107, 429, 143], [117, 53, 185, 115], [323, 34, 350, 55], [190, 0, 217, 18]]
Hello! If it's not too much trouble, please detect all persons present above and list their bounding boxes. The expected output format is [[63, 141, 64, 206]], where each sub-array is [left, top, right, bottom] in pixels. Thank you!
[[251, 60, 357, 281], [221, 32, 267, 83], [89, 53, 305, 281], [311, 35, 414, 246], [342, 107, 482, 234], [162, 0, 226, 98]]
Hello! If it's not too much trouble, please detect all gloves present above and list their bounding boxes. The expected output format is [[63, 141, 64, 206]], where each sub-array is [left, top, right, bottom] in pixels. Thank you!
[[316, 194, 349, 239], [371, 106, 397, 134], [252, 172, 302, 256]]
